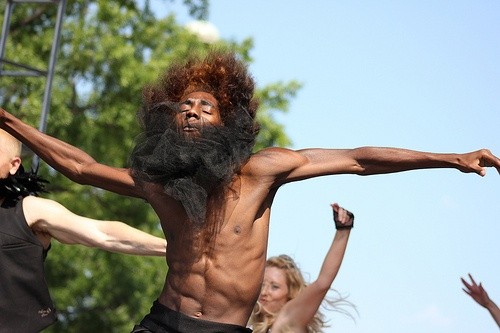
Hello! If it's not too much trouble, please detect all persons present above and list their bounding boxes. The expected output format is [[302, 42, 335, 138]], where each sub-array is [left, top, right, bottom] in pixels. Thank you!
[[0, 130, 166, 333], [1, 43, 500, 333], [246, 202, 354, 333], [460, 272, 500, 327]]
